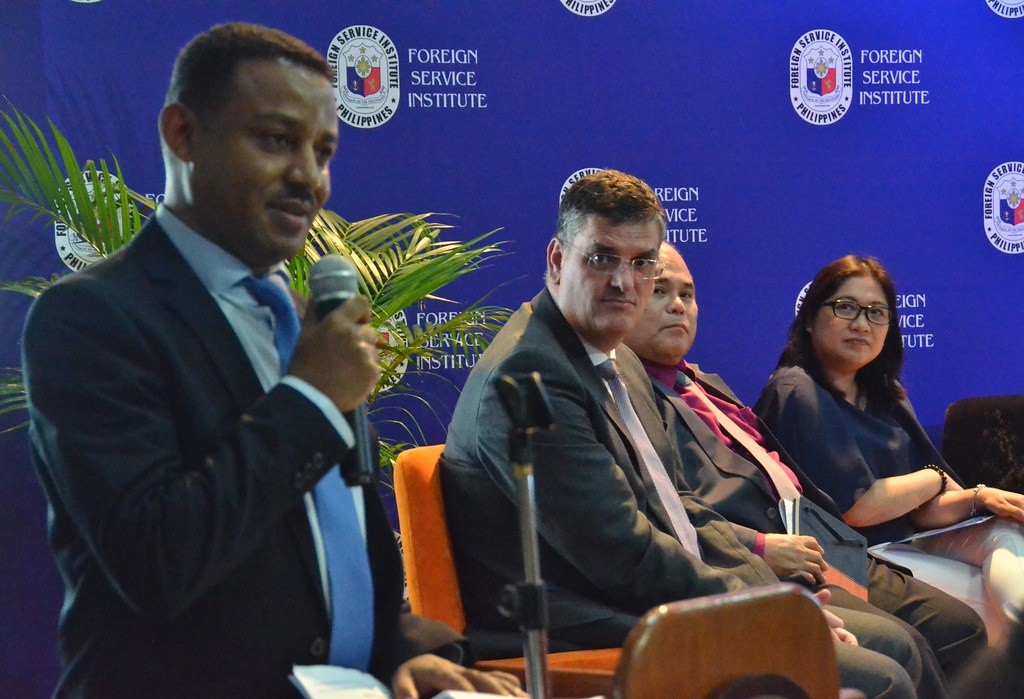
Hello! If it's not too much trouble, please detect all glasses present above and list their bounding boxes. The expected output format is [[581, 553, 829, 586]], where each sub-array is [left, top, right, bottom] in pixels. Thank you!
[[819, 300, 892, 325], [563, 239, 665, 280]]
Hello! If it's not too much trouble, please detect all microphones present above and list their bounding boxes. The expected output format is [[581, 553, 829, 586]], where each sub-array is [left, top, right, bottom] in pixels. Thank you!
[[306, 254, 371, 486]]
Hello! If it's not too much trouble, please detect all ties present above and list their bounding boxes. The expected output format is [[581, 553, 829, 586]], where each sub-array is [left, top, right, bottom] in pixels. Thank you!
[[231, 273, 373, 674], [676, 370, 800, 503], [594, 358, 702, 565]]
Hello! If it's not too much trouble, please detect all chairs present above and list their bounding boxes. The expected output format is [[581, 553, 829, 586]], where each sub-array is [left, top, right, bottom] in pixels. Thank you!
[[393, 444, 623, 699]]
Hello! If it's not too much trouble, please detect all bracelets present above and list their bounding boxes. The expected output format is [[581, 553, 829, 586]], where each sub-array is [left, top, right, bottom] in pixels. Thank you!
[[970, 484, 986, 517], [922, 464, 947, 496]]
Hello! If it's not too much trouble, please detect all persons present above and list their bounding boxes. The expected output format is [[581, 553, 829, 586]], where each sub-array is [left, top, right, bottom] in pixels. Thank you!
[[436, 169, 1024, 699], [24, 20, 532, 699]]
